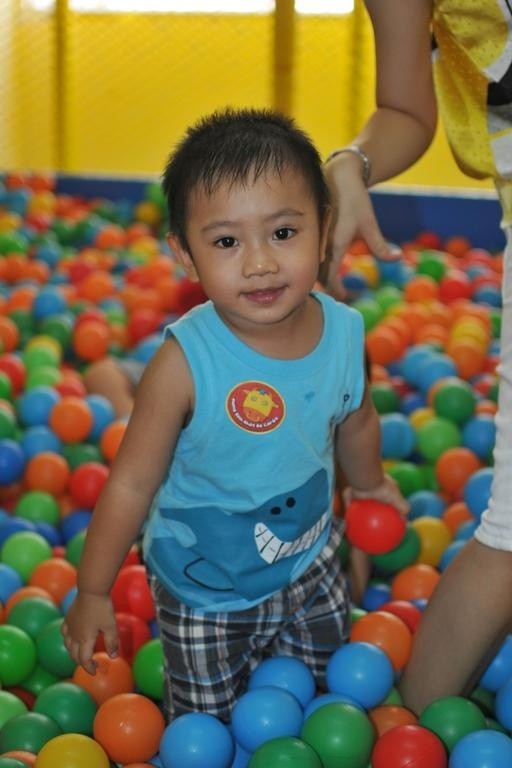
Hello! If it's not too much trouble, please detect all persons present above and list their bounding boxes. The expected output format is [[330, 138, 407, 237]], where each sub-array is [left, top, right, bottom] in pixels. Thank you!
[[47, 107, 409, 729], [320, 0, 512, 708]]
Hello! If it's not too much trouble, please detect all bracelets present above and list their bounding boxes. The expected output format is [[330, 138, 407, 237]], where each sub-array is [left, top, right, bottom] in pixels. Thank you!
[[326, 144, 372, 183]]
